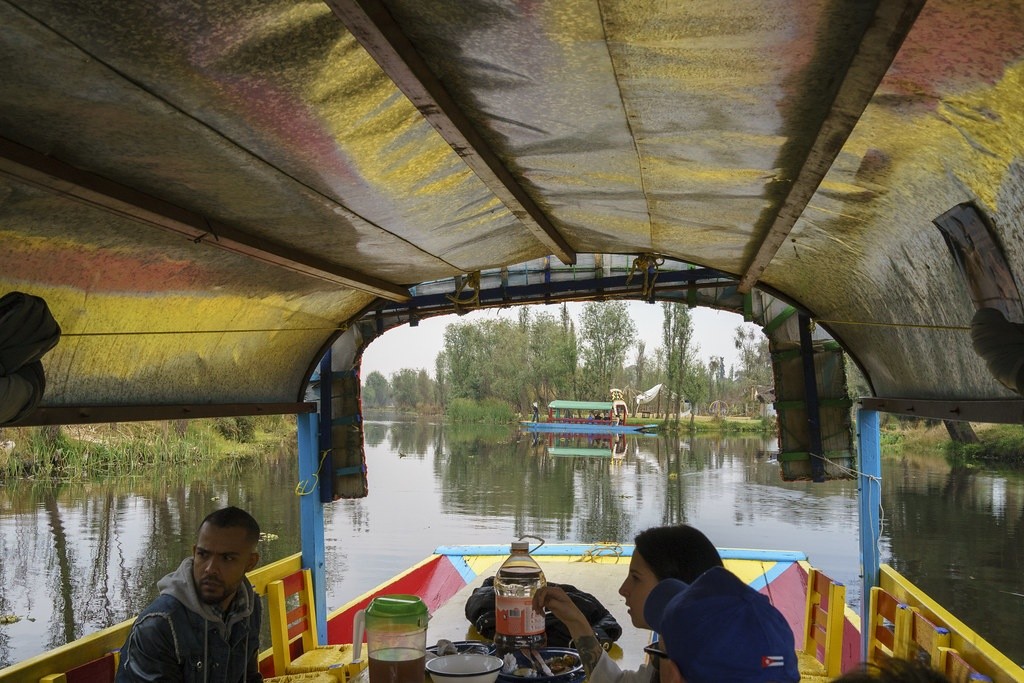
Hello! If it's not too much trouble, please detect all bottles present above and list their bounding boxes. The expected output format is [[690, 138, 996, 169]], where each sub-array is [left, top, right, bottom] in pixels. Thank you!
[[494, 541, 547, 660]]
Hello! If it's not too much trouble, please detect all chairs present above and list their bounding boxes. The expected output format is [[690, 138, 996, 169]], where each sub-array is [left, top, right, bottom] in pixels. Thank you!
[[795, 567, 847, 677], [936, 646, 994, 683], [262, 664, 346, 683], [799, 585, 909, 683], [902, 606, 951, 669], [267, 567, 368, 677]]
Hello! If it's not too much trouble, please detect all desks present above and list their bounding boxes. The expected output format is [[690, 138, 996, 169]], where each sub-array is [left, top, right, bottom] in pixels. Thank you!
[[344, 559, 654, 683]]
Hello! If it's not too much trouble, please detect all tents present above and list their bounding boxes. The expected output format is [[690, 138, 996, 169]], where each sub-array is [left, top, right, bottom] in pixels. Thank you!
[[633, 382, 685, 417]]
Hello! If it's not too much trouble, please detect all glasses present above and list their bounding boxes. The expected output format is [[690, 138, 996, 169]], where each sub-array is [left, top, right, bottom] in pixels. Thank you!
[[644, 642, 669, 671]]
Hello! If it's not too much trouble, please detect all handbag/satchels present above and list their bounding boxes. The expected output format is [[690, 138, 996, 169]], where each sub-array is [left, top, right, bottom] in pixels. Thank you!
[[465, 576, 623, 652]]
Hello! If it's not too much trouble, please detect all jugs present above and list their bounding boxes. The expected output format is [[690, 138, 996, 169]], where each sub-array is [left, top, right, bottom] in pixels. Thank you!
[[353, 594, 433, 683]]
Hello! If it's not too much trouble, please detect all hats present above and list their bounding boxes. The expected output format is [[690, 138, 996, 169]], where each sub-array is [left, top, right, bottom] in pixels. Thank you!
[[644, 568, 800, 683]]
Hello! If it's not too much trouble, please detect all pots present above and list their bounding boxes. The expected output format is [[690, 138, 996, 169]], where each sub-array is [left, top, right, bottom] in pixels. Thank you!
[[426, 640, 497, 660], [500, 649, 584, 683]]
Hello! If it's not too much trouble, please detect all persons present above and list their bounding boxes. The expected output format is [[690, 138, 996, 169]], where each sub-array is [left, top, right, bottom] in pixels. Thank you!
[[641, 565, 801, 683], [588, 433, 620, 449], [826, 653, 950, 683], [531, 523, 726, 683], [110, 506, 266, 683], [530, 432, 539, 449], [530, 405, 540, 422], [586, 411, 620, 426]]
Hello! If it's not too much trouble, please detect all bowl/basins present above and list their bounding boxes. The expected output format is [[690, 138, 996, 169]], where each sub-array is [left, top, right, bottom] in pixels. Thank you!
[[425, 654, 504, 683]]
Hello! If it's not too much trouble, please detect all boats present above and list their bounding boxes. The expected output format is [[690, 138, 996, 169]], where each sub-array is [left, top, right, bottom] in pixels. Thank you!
[[518, 426, 658, 460], [519, 399, 659, 431]]
